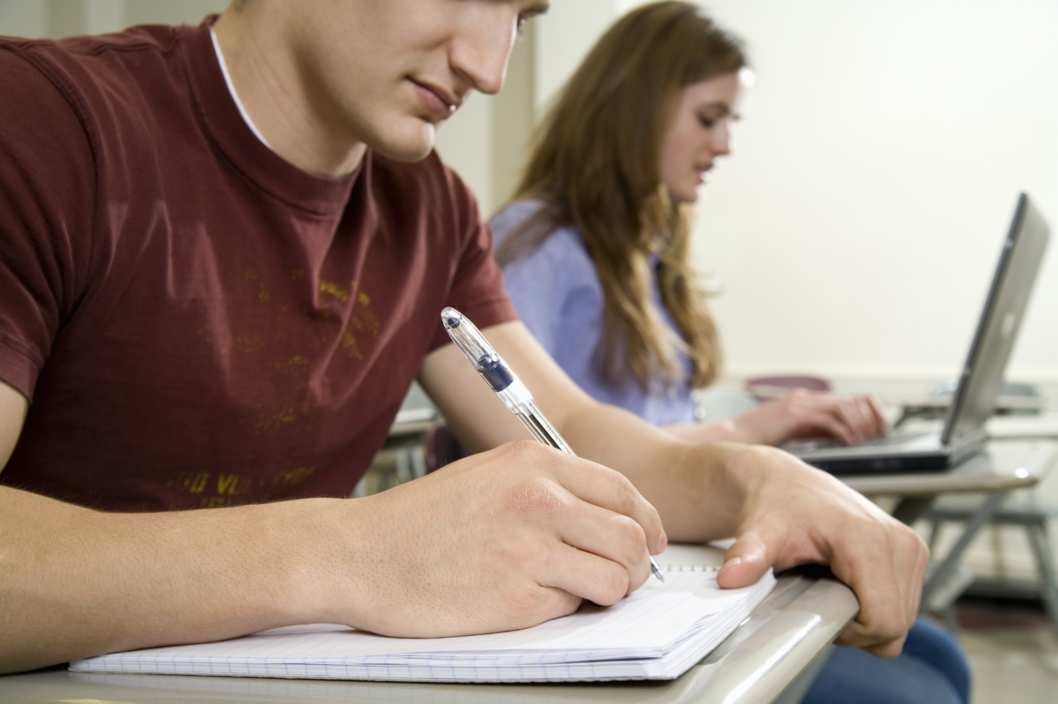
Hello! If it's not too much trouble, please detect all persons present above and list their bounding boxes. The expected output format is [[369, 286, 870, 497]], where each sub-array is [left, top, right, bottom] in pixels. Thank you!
[[486, 1, 971, 704], [0, 0, 932, 703]]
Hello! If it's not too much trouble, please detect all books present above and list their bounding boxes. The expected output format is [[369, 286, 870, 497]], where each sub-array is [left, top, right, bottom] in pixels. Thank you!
[[67, 531, 780, 682]]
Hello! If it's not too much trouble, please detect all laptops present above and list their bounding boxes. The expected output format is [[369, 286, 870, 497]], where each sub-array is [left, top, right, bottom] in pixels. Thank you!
[[777, 192, 1052, 474]]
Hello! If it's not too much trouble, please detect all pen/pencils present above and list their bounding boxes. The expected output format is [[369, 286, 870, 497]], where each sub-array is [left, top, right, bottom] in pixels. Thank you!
[[442, 308, 669, 581]]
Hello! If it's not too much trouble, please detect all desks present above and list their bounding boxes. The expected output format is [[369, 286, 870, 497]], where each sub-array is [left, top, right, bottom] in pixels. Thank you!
[[354, 412, 446, 496], [0, 573, 860, 704], [824, 437, 1057, 607]]
